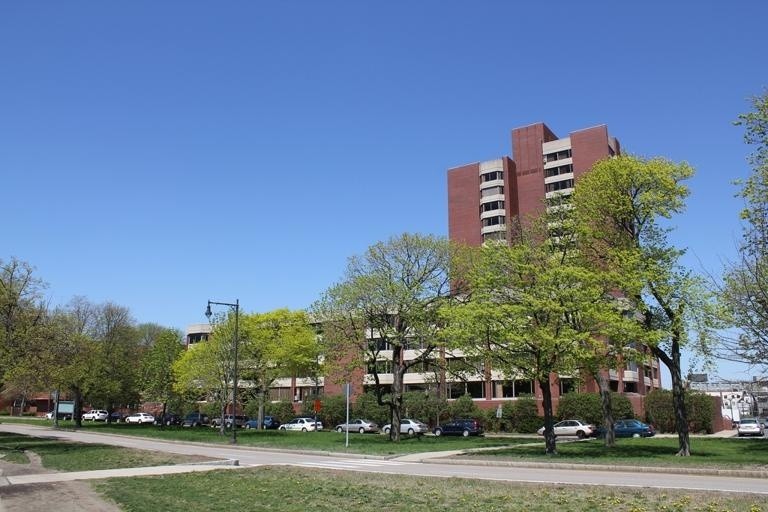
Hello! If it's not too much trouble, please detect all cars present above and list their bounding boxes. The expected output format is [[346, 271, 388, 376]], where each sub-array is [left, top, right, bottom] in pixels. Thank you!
[[277, 416, 488, 438], [732, 416, 768, 436], [538, 418, 597, 438], [592, 420, 657, 439], [43, 400, 283, 430]]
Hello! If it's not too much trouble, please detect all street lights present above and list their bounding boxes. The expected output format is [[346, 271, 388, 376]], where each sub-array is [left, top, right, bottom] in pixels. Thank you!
[[205, 299, 240, 443]]
